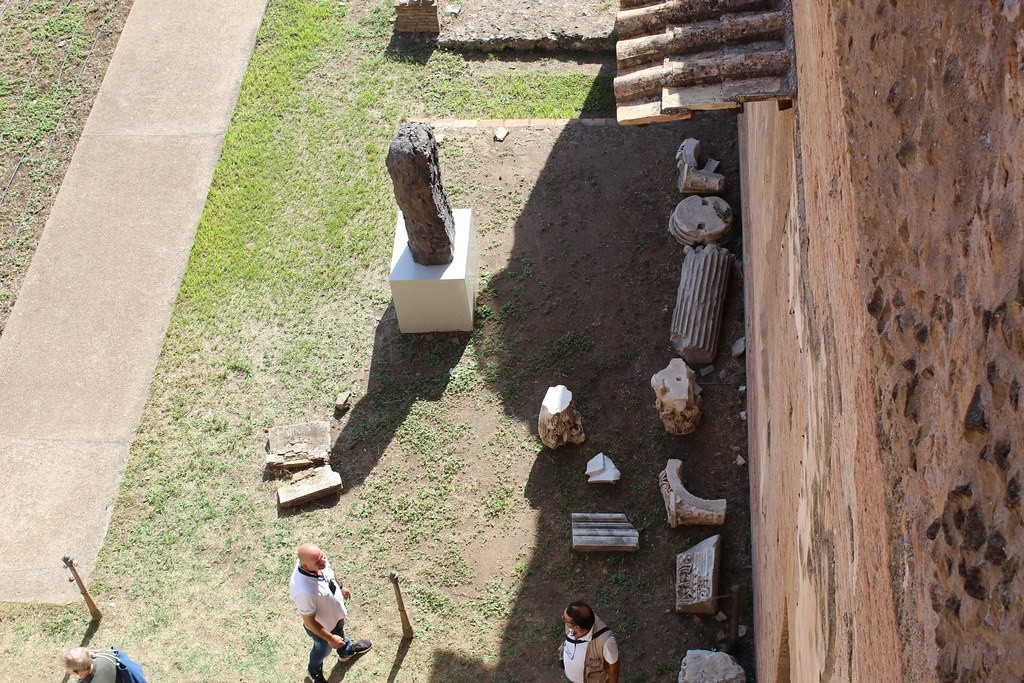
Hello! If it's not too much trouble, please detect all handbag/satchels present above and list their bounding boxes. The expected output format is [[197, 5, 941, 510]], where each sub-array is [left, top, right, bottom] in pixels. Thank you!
[[560, 659, 564, 670], [110, 646, 147, 683]]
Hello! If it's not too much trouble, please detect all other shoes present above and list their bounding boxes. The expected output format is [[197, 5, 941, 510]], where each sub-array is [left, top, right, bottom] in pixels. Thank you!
[[306, 666, 327, 683], [338, 640, 372, 662]]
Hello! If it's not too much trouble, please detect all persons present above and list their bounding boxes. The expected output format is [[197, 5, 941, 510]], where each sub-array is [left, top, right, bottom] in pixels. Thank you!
[[557, 601, 620, 683], [289, 543, 372, 683], [61, 647, 124, 683]]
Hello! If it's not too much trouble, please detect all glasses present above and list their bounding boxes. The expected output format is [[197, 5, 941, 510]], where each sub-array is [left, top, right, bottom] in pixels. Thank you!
[[562, 616, 573, 624]]
